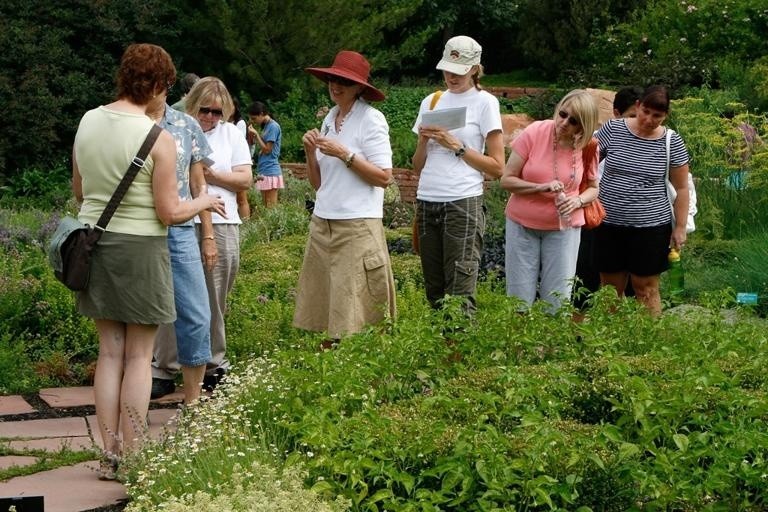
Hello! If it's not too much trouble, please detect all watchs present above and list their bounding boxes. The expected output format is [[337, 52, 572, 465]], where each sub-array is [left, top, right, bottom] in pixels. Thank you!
[[455, 143, 467, 157], [346, 153, 354, 166]]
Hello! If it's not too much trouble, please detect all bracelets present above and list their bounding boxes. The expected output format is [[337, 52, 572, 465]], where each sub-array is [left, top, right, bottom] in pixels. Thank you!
[[347, 154, 356, 168], [578, 196, 584, 208]]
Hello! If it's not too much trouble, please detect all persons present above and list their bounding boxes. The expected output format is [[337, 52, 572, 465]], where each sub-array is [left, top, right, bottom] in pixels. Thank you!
[[71, 45, 230, 479], [146, 70, 255, 409], [291, 50, 398, 350], [407, 35, 510, 359], [244, 102, 283, 205], [500, 88, 598, 313], [718, 109, 763, 190], [577, 86, 698, 313]]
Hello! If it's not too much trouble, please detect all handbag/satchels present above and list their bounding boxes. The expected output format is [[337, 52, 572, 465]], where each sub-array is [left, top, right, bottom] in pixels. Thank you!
[[580, 173, 607, 228], [49, 216, 97, 290], [665, 170, 698, 232]]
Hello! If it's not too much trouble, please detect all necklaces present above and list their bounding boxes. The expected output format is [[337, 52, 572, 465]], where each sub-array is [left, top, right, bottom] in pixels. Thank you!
[[552, 128, 576, 189]]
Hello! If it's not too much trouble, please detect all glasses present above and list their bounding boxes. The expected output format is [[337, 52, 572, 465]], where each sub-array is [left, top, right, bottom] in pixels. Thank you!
[[557, 110, 577, 126], [199, 107, 221, 115]]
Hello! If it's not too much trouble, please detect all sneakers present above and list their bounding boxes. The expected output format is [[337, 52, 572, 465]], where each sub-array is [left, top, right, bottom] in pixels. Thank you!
[[202, 370, 225, 392], [149, 378, 175, 399]]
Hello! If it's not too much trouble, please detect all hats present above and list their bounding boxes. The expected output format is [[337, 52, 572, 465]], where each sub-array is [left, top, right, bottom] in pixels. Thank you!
[[306, 51, 385, 102], [436, 36, 483, 76]]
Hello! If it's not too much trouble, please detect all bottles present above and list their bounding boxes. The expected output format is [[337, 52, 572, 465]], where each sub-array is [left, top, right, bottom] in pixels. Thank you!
[[554, 190, 573, 232], [668, 248, 684, 294]]
